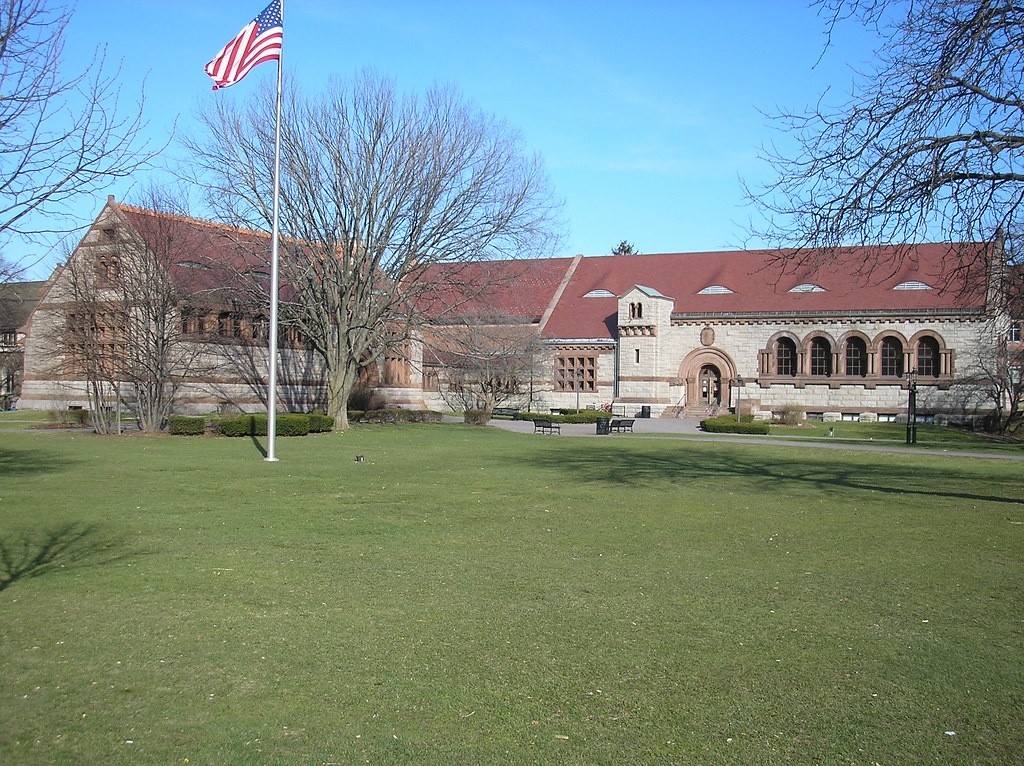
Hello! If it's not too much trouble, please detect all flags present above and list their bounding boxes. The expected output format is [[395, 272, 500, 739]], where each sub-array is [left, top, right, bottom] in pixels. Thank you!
[[204, 0, 284, 91]]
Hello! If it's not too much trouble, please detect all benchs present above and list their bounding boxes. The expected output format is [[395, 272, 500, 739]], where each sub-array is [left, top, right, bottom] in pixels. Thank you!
[[532, 418, 561, 435], [608, 420, 635, 433], [491, 408, 521, 421]]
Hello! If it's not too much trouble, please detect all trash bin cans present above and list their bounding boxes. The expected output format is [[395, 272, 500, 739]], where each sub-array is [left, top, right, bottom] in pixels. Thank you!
[[642, 406, 651, 418], [596, 416, 610, 436], [550, 408, 565, 416]]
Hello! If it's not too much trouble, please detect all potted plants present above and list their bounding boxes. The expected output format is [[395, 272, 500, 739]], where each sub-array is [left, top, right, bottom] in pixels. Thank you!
[[783, 404, 807, 420]]
